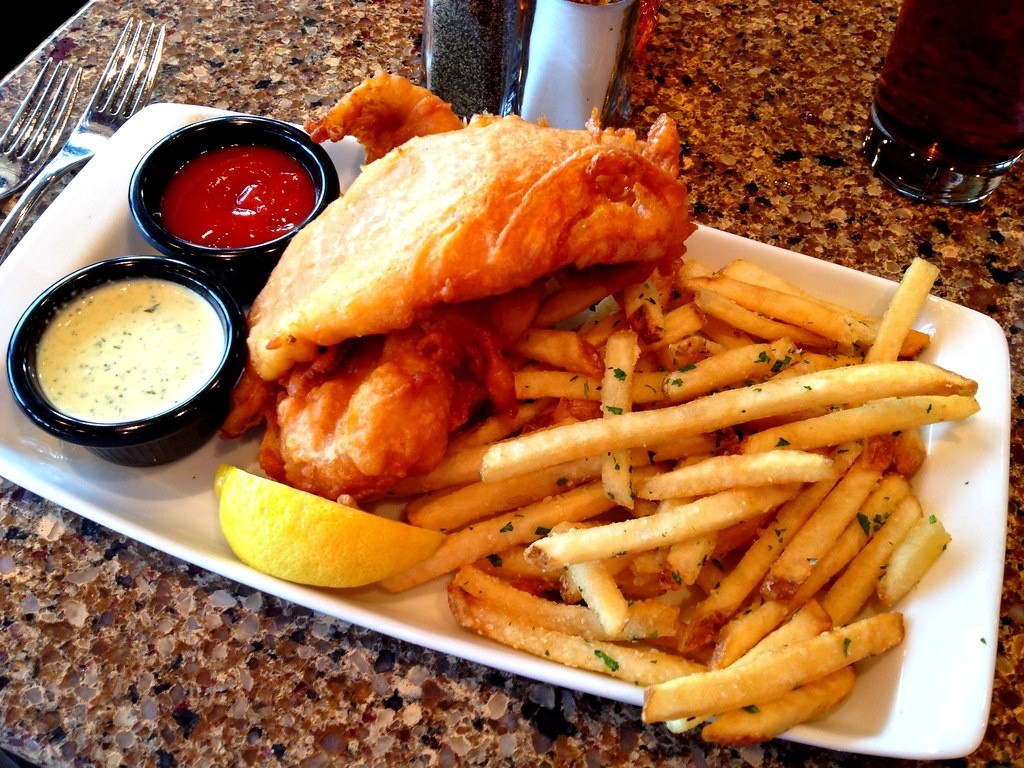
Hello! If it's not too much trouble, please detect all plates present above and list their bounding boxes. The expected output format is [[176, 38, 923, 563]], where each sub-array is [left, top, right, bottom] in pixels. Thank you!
[[1, 101, 1012, 760]]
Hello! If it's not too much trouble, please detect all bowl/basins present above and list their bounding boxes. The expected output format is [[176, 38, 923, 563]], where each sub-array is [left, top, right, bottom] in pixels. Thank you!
[[4, 255, 245, 468], [129, 116, 339, 312]]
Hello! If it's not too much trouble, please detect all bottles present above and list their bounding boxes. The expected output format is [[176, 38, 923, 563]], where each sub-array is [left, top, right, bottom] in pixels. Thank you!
[[416, 0, 528, 121], [510, 2, 653, 139]]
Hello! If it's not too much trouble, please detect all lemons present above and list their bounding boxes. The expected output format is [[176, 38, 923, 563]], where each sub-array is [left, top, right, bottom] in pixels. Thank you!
[[214, 463, 449, 591]]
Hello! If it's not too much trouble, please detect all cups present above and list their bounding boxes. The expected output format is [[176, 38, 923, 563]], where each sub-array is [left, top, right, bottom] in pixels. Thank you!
[[861, 1, 1024, 203]]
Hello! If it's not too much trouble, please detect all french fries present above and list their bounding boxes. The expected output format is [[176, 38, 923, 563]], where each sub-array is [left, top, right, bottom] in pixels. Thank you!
[[340, 253, 980, 746]]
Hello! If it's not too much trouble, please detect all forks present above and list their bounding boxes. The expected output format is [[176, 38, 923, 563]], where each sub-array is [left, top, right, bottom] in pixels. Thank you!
[[1, 16, 166, 249], [1, 57, 82, 200]]
